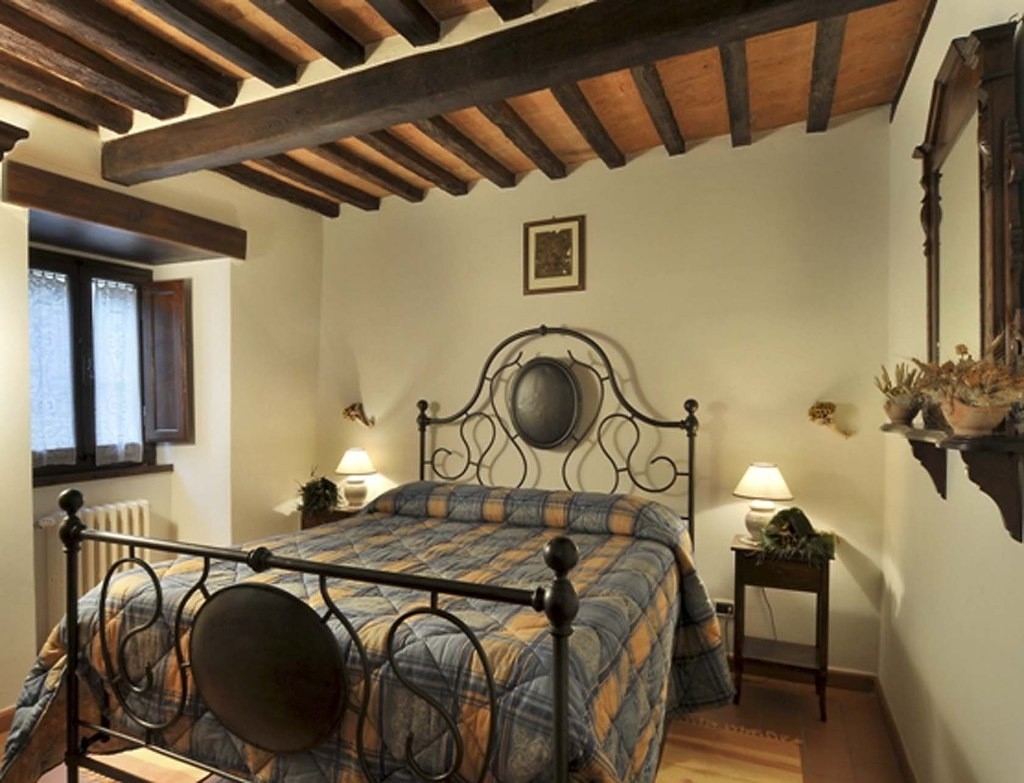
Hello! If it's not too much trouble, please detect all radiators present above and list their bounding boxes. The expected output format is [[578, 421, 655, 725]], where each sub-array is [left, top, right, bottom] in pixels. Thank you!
[[40, 498, 151, 631]]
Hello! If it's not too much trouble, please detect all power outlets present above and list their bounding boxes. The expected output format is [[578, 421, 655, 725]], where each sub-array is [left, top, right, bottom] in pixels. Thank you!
[[713, 599, 735, 618]]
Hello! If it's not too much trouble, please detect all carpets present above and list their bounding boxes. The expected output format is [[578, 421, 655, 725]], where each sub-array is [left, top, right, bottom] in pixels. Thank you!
[[653, 711, 802, 783], [84, 744, 212, 783]]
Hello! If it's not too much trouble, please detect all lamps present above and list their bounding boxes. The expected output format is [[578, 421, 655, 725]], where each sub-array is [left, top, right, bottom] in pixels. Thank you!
[[732, 463, 793, 545], [335, 447, 377, 507]]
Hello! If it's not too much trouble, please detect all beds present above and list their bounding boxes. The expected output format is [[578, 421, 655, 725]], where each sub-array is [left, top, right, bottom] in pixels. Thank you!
[[0, 323, 740, 783]]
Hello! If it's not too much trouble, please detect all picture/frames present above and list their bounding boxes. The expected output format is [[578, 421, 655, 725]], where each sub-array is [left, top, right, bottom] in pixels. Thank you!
[[523, 214, 587, 295]]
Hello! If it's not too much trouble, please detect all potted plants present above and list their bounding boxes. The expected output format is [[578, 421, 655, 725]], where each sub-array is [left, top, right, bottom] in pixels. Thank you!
[[873, 359, 930, 429], [911, 342, 1023, 436]]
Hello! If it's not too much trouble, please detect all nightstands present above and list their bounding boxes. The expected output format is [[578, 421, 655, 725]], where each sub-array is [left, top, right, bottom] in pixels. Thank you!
[[301, 510, 356, 529], [732, 533, 836, 723]]
